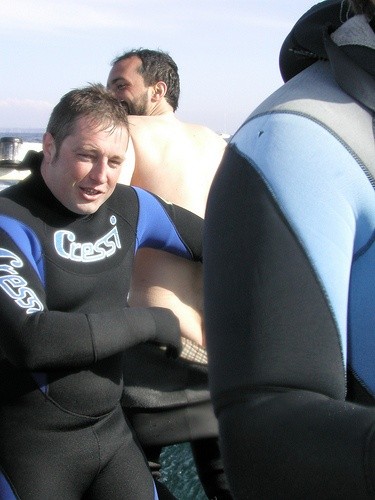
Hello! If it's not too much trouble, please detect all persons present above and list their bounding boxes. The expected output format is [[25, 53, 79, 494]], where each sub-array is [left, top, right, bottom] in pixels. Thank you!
[[201, 0, 374, 500], [1, 49, 234, 500]]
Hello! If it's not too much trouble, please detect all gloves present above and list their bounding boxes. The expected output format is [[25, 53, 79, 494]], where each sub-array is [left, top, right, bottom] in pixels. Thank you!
[[86, 305, 182, 363]]
